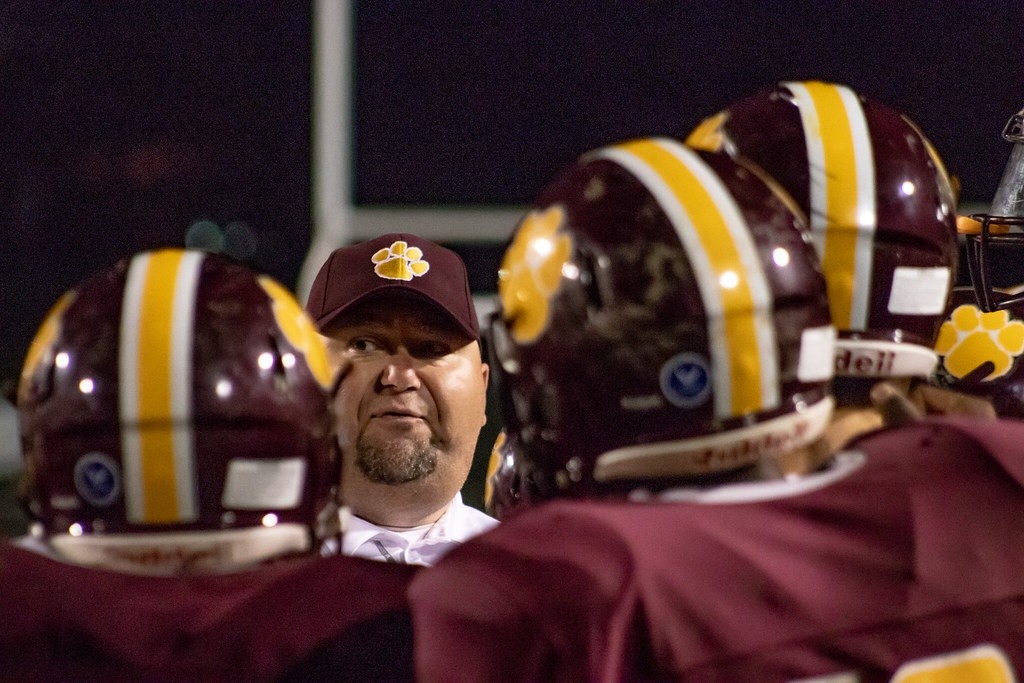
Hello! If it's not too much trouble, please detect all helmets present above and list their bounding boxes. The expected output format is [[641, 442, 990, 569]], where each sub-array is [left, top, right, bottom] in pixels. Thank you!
[[965, 109, 1024, 326], [687, 80, 958, 396], [489, 138, 836, 517], [18, 249, 337, 576]]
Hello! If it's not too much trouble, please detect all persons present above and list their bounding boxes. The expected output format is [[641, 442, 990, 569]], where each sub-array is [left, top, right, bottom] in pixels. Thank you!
[[0, 75, 1024, 683]]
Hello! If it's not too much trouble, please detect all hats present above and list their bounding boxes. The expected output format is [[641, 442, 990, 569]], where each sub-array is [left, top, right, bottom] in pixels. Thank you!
[[305, 233, 482, 354]]
